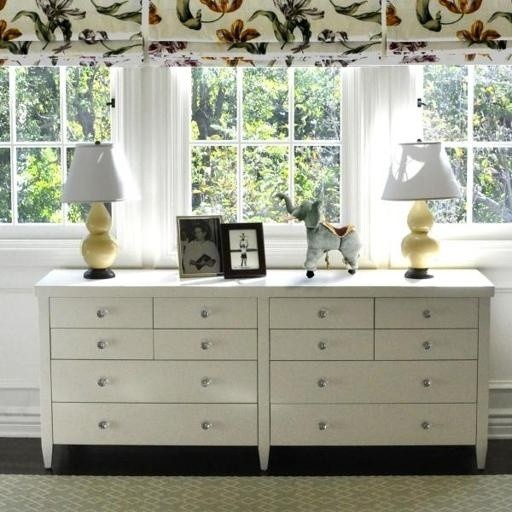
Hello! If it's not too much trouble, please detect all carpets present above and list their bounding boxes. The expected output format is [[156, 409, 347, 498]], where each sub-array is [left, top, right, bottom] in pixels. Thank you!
[[0, 473, 511, 512]]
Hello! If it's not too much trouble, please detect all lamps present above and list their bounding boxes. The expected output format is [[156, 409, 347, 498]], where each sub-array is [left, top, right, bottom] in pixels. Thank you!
[[380, 139, 461, 279], [60, 143, 127, 279]]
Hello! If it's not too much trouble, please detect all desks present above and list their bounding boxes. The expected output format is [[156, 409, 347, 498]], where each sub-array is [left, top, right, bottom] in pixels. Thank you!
[[34, 267, 495, 472]]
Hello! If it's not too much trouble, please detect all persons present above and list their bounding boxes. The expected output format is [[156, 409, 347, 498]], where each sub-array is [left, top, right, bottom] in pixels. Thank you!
[[183, 225, 221, 273], [240, 233, 249, 266]]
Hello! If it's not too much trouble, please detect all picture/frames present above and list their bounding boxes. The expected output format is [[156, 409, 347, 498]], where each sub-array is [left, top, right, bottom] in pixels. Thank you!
[[175, 215, 226, 279], [220, 222, 267, 278]]
